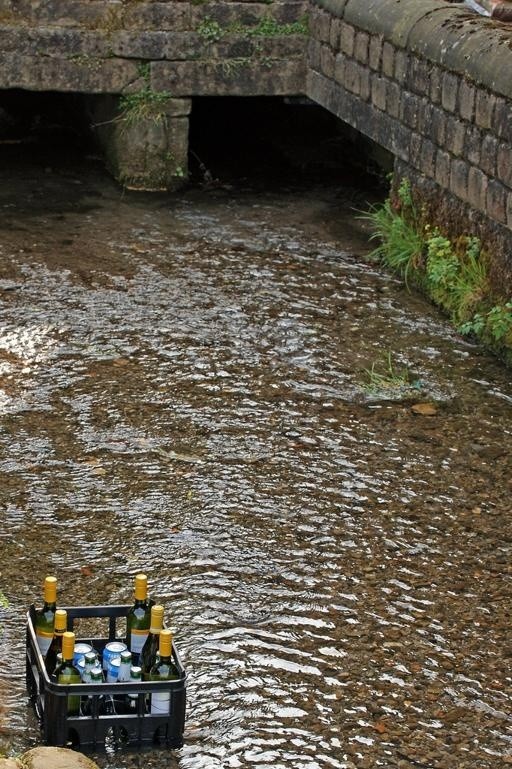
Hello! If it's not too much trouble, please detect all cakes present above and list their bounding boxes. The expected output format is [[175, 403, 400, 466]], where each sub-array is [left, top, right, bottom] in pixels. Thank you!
[[103, 642, 128, 682], [73, 644, 102, 677]]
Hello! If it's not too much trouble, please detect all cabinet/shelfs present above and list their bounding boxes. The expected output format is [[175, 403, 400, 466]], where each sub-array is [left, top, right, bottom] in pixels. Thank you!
[[33, 572, 182, 722]]
